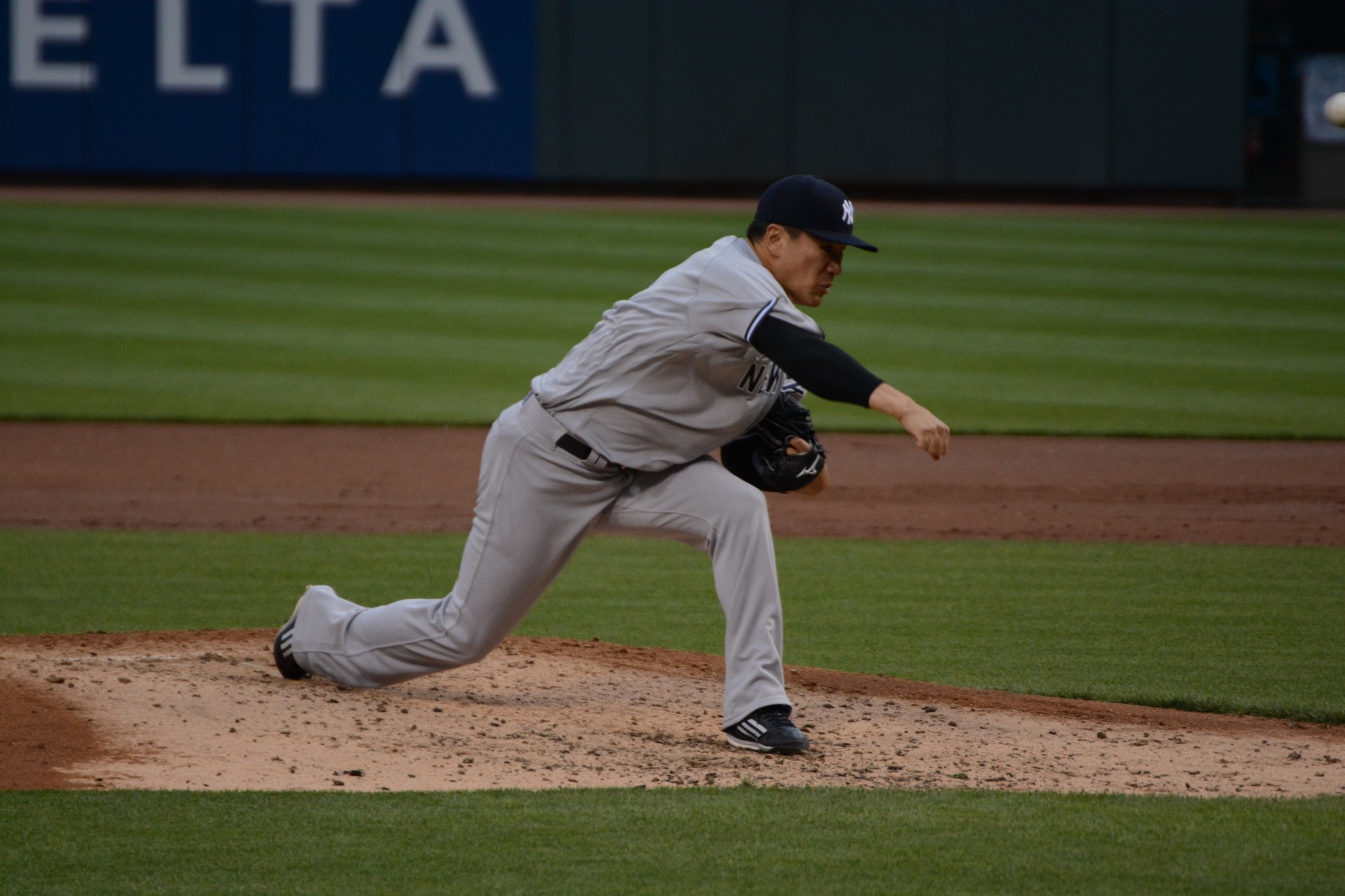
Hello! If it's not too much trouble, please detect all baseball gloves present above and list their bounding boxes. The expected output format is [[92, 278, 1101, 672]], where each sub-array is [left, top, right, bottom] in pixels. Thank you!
[[720, 394, 825, 495]]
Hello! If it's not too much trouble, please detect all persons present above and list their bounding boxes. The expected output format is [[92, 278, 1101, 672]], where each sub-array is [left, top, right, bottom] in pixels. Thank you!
[[274, 175, 951, 753]]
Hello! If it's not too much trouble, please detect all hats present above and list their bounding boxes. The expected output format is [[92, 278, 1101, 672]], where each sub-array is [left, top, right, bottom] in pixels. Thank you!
[[755, 174, 880, 252]]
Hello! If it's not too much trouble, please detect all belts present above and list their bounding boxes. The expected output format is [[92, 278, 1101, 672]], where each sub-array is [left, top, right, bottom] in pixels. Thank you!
[[556, 434, 646, 474]]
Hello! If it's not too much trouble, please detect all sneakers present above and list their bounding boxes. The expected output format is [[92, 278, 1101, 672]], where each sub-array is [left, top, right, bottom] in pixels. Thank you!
[[725, 703, 810, 753], [271, 585, 308, 680]]
